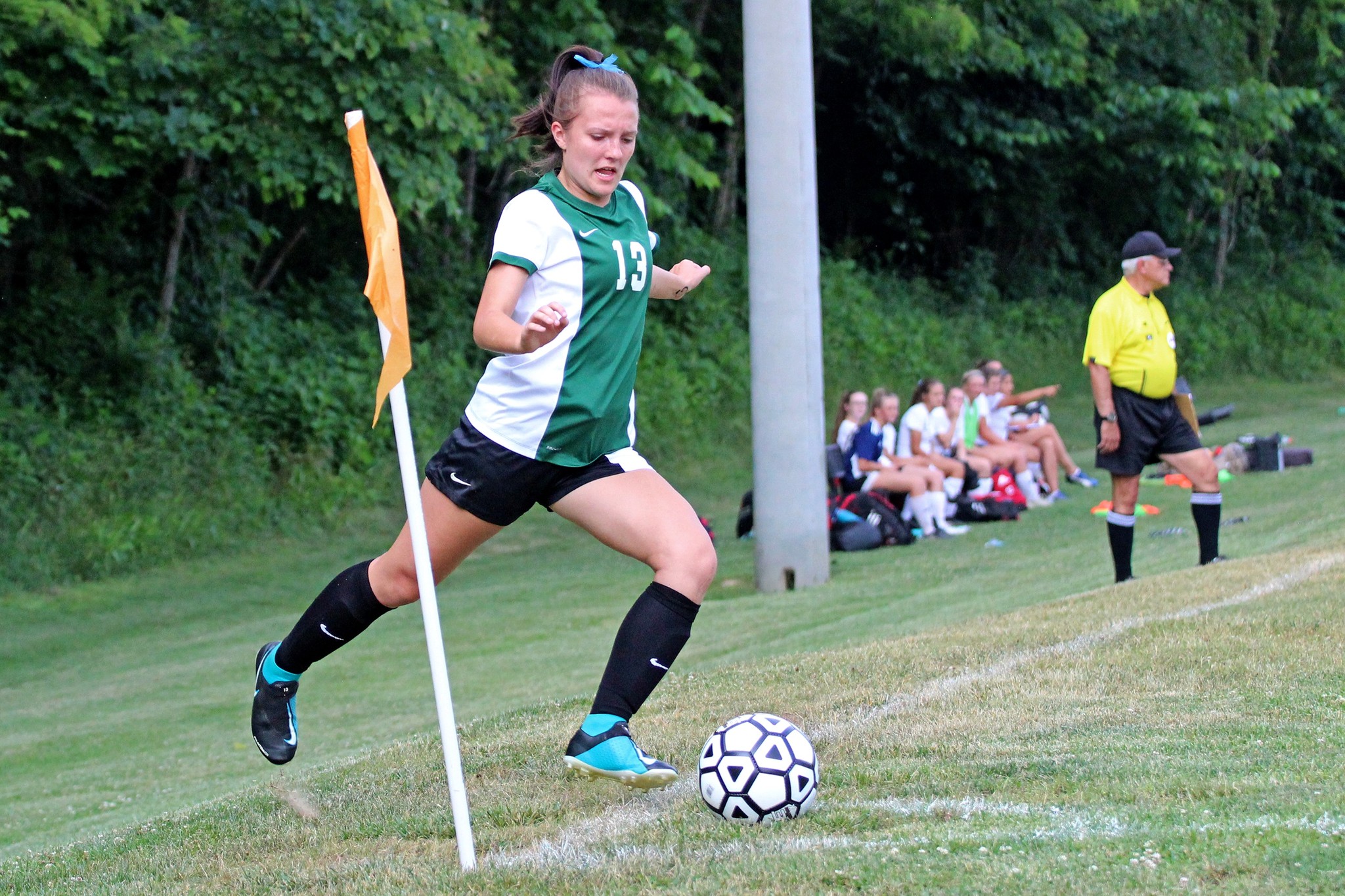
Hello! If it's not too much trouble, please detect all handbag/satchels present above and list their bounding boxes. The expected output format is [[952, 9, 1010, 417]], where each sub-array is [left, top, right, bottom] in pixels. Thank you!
[[1236, 431, 1286, 472]]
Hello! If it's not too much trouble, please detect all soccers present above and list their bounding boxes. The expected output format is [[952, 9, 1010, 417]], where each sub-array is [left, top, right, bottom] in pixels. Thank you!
[[696, 711, 819, 828]]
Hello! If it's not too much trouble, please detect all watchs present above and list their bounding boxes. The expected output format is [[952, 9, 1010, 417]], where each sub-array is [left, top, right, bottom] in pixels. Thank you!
[[1099, 410, 1119, 423]]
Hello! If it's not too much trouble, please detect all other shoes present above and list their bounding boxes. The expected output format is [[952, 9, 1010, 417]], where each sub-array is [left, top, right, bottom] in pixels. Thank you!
[[1047, 489, 1070, 502], [1065, 467, 1099, 488], [1025, 493, 1051, 508]]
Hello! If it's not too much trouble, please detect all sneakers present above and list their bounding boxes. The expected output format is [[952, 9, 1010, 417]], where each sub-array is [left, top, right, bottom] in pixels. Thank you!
[[251, 641, 301, 765], [564, 718, 679, 792]]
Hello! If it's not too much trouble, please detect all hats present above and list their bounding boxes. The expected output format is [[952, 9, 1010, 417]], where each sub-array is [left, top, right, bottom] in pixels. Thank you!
[[1122, 230, 1183, 259]]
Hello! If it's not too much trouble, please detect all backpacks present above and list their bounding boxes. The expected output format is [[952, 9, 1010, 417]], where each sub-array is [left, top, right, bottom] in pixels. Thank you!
[[992, 465, 1028, 511], [840, 491, 915, 545], [956, 497, 1020, 522], [830, 519, 885, 552]]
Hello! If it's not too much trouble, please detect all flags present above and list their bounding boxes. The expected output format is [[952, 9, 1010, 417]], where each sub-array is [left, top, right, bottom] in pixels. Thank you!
[[345, 117, 416, 431]]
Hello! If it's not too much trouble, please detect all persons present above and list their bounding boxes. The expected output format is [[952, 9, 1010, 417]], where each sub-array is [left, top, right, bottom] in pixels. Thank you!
[[1081, 231, 1222, 583], [247, 45, 718, 786], [834, 357, 1098, 537]]
